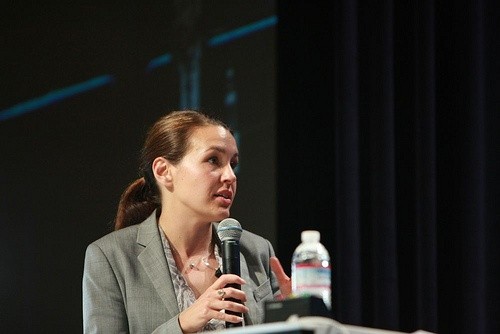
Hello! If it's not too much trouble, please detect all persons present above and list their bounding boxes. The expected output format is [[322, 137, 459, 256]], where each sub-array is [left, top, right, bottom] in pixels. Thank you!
[[82, 110, 291, 334]]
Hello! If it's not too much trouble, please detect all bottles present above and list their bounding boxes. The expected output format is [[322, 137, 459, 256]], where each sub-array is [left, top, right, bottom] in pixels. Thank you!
[[291, 230, 332, 311]]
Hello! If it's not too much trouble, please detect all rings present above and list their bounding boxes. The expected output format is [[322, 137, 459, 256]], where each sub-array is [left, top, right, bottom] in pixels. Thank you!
[[217, 288, 226, 299]]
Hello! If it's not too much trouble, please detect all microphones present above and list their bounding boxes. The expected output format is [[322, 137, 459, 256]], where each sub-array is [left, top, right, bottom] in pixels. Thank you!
[[217, 218, 244, 328]]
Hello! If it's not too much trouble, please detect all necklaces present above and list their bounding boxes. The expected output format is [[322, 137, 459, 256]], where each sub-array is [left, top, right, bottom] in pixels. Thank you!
[[169, 241, 215, 269]]
